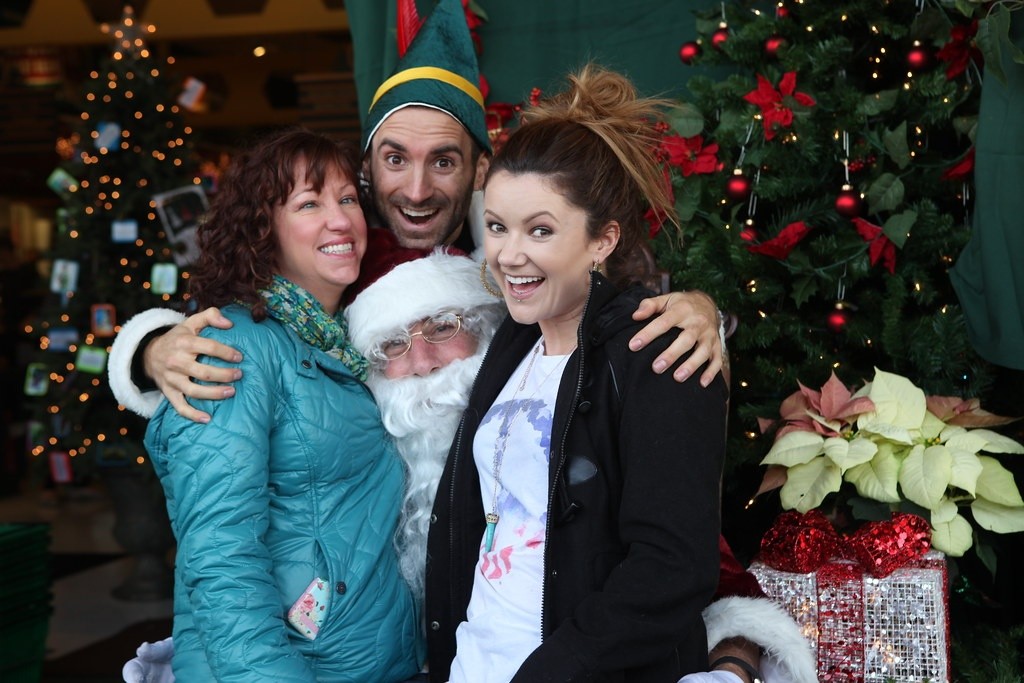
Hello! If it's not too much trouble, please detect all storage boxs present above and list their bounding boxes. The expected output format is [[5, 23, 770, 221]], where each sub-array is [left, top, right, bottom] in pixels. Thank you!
[[746, 549, 953, 683]]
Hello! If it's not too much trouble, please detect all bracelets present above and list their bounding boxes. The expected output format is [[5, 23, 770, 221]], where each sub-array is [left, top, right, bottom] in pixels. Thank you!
[[710, 656, 765, 683]]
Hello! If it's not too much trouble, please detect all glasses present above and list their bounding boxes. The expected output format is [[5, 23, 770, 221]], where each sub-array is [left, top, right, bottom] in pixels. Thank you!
[[372, 313, 466, 361]]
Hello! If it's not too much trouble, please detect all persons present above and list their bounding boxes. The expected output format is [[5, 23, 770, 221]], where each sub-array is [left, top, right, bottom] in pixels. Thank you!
[[106, 106, 742, 425], [423, 63, 731, 683], [142, 131, 425, 683], [122, 226, 816, 683]]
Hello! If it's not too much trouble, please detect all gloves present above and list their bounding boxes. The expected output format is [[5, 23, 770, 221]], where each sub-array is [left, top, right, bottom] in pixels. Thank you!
[[678, 670, 746, 683], [121, 637, 177, 683]]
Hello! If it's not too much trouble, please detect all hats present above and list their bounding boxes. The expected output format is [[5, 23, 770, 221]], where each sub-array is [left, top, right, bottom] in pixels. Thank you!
[[362, 0, 494, 157], [339, 228, 503, 360]]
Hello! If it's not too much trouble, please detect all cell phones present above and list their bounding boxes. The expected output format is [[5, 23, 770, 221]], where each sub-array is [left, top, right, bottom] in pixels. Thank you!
[[287, 577, 330, 640]]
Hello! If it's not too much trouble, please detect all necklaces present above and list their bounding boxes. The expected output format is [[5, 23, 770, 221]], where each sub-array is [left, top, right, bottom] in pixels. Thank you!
[[485, 332, 579, 552]]
